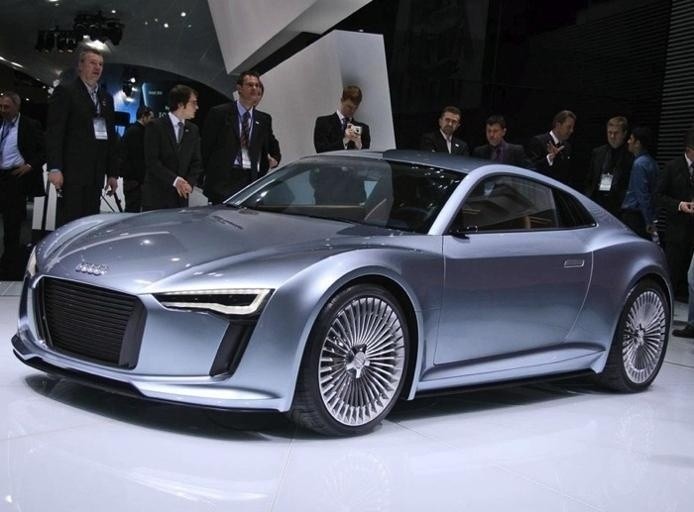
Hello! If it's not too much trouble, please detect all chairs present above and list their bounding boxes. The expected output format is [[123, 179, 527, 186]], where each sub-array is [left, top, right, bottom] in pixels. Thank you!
[[361, 170, 533, 231]]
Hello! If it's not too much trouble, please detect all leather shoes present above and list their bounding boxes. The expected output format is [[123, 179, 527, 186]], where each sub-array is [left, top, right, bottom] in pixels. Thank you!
[[672, 324, 694, 338]]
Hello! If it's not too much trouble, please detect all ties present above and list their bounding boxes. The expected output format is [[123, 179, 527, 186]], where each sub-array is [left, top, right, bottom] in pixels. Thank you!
[[0, 120, 16, 168], [177, 122, 184, 142], [445, 134, 451, 152], [237, 112, 251, 170], [342, 117, 350, 149]]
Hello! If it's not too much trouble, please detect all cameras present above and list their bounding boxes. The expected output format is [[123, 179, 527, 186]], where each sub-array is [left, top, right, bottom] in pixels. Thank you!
[[351, 126, 363, 135]]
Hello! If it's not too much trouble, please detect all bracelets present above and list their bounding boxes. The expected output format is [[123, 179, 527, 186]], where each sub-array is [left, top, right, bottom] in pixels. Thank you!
[[26, 164, 31, 168]]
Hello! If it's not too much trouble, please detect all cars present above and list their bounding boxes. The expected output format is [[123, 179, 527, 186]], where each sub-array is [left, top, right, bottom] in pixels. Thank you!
[[10, 150, 674, 437]]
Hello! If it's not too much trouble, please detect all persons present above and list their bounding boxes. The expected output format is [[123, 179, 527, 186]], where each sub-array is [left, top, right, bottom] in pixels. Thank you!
[[313, 85, 371, 205], [120, 103, 155, 213], [471, 111, 532, 170], [581, 116, 636, 226], [668, 250, 693, 338], [0, 89, 48, 281], [616, 126, 661, 243], [652, 132, 694, 303], [44, 48, 124, 232], [252, 80, 280, 173], [199, 70, 268, 205], [418, 106, 471, 161], [530, 110, 579, 188], [139, 83, 204, 211]]
